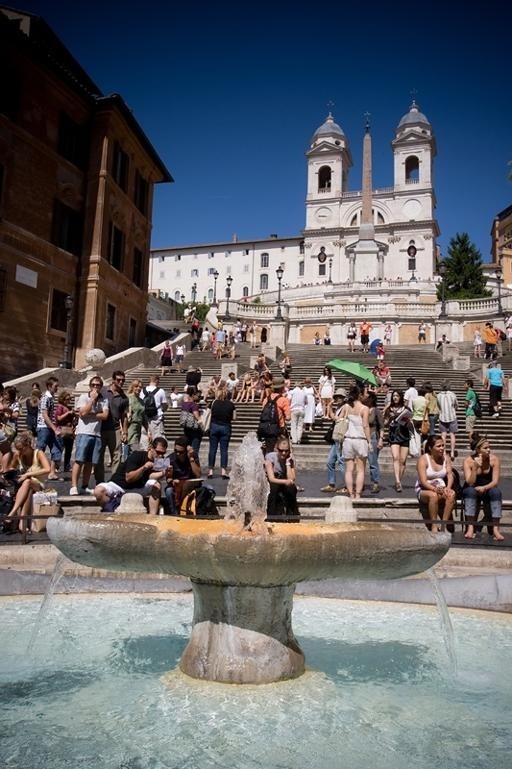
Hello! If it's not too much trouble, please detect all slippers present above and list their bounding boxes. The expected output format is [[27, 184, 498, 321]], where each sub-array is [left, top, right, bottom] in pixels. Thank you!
[[47, 467, 74, 481]]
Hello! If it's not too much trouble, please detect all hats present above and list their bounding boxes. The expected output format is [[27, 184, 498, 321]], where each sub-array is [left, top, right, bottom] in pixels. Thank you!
[[333, 388, 346, 400], [271, 376, 286, 388]]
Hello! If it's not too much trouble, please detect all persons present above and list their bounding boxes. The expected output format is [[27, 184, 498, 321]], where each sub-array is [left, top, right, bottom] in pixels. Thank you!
[[483, 360, 506, 419], [359, 319, 373, 353], [347, 321, 357, 352], [314, 331, 322, 345], [323, 331, 331, 345], [320, 379, 478, 501], [376, 342, 380, 350], [461, 432, 505, 541], [417, 319, 426, 344], [158, 302, 269, 377], [0, 350, 319, 535], [317, 366, 337, 420], [384, 324, 392, 344], [370, 361, 391, 387], [414, 434, 456, 533], [437, 335, 450, 348], [472, 313, 512, 361], [376, 340, 386, 360]]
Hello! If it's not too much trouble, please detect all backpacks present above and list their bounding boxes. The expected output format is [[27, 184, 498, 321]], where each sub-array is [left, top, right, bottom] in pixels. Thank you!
[[180, 486, 219, 516], [141, 387, 161, 417], [257, 394, 283, 441], [472, 389, 483, 417]]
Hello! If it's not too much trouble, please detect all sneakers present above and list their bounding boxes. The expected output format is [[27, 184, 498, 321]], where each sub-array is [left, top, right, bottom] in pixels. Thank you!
[[70, 486, 93, 496], [492, 407, 503, 417], [0, 520, 13, 533], [371, 485, 379, 493], [207, 475, 229, 479], [395, 482, 403, 492], [320, 486, 349, 494], [296, 484, 305, 492]]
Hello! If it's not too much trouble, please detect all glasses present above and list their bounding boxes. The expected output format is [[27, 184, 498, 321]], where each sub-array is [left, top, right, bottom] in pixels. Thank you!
[[90, 383, 100, 387], [117, 378, 125, 382], [154, 450, 167, 455], [277, 447, 291, 452]]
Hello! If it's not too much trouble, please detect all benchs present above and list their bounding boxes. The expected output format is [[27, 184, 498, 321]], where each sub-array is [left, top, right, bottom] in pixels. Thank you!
[[0, 496, 512, 543]]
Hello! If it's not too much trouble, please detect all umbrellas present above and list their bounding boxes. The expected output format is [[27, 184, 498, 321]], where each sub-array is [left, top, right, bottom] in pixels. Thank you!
[[370, 338, 381, 352], [325, 359, 379, 387]]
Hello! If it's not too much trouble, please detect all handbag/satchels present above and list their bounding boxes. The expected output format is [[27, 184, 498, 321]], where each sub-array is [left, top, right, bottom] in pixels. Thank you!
[[285, 365, 292, 373], [103, 491, 122, 512], [409, 431, 422, 457], [324, 418, 348, 444], [316, 398, 324, 416], [60, 426, 74, 437], [31, 489, 61, 532], [421, 421, 430, 434], [500, 331, 506, 340], [179, 411, 200, 430], [198, 407, 212, 432]]
